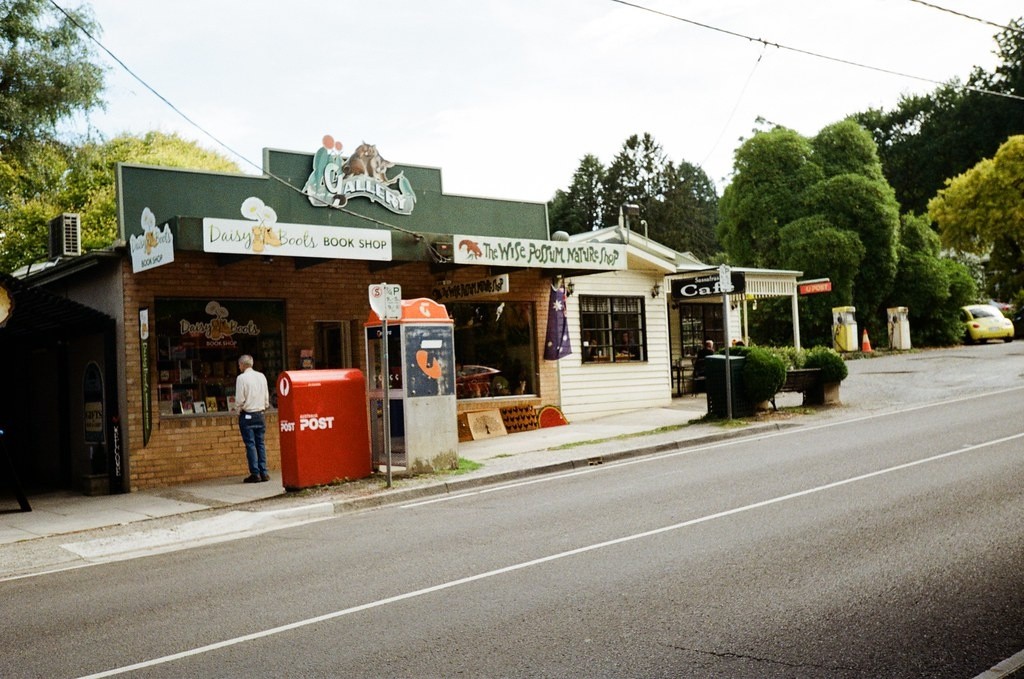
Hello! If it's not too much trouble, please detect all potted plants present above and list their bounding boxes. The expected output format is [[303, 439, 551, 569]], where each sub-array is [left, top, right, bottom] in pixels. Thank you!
[[803, 345, 849, 408], [710, 345, 786, 414], [80, 436, 110, 497]]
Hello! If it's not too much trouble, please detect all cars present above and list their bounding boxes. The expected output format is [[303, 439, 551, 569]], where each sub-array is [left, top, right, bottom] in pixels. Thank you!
[[988, 298, 1014, 312], [957, 304, 1015, 344]]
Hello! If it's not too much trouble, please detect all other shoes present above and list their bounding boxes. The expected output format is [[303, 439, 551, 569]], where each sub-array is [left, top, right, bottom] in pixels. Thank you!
[[260, 473, 270, 481], [244, 472, 262, 482]]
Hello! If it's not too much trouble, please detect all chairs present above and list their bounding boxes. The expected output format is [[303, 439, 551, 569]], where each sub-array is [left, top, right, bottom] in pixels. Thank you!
[[672, 357, 707, 399]]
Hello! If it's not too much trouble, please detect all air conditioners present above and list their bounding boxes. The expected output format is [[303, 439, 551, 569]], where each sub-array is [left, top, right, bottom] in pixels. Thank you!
[[48, 212, 81, 260]]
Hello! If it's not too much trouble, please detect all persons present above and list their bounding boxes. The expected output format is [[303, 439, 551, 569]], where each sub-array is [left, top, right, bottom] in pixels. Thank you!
[[735, 341, 746, 347], [234, 355, 271, 483], [693, 340, 715, 376]]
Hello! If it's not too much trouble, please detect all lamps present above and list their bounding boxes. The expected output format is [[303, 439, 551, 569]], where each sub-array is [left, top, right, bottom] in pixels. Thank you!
[[566, 279, 575, 296], [731, 299, 738, 310], [672, 300, 679, 310], [651, 280, 660, 298], [752, 299, 757, 311]]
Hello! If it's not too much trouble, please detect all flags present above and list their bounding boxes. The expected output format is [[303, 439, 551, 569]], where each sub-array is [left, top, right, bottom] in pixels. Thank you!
[[543, 275, 572, 360]]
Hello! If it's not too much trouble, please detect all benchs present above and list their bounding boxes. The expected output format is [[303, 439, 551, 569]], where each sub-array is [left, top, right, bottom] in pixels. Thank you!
[[769, 368, 821, 411]]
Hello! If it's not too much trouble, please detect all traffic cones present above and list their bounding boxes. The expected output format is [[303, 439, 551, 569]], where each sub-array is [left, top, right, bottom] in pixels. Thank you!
[[862, 329, 873, 352]]
